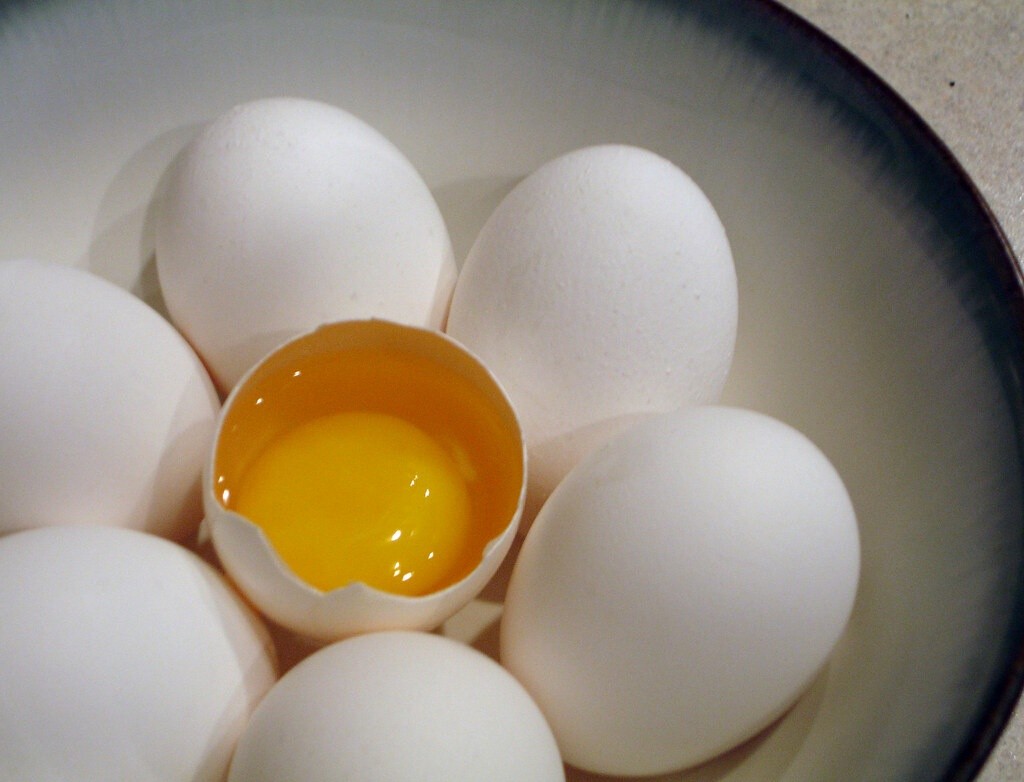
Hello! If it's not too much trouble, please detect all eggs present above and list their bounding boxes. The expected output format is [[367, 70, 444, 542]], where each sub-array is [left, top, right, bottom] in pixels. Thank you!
[[1, 97, 864, 782]]
